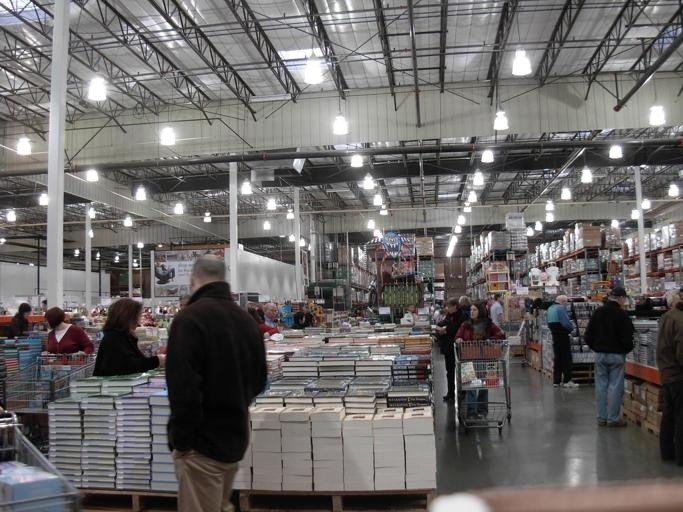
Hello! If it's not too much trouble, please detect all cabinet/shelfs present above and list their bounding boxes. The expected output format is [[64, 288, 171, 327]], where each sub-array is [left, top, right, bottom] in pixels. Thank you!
[[366, 238, 437, 321], [464, 246, 532, 357], [622, 235, 683, 308], [528, 240, 628, 302], [309, 234, 381, 314]]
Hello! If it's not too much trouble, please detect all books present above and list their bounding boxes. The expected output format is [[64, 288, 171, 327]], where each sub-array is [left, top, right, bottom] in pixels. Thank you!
[[253, 324, 438, 492], [231, 406, 252, 490], [49, 369, 178, 492]]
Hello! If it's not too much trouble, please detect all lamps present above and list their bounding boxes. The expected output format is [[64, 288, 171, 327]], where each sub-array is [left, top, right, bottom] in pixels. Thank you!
[[467, 189, 478, 203], [372, 194, 384, 207], [358, 172, 379, 192], [639, 193, 653, 211], [123, 212, 134, 228], [262, 215, 272, 231], [534, 218, 543, 231], [510, 10, 535, 78], [666, 180, 680, 198], [630, 207, 644, 221], [579, 151, 595, 187], [647, 70, 669, 129], [480, 146, 496, 165], [348, 151, 366, 170], [558, 183, 575, 202], [491, 76, 512, 133], [15, 134, 34, 157], [285, 206, 295, 220], [452, 223, 463, 233], [239, 161, 256, 197], [455, 212, 467, 226], [133, 183, 148, 202], [606, 143, 627, 160], [525, 223, 535, 237], [544, 198, 556, 211], [461, 204, 472, 213], [471, 158, 486, 187], [156, 100, 182, 148], [265, 196, 277, 212], [295, 28, 328, 87], [327, 93, 353, 139], [84, 166, 99, 184], [202, 209, 213, 223], [381, 204, 387, 210], [544, 211, 556, 223], [365, 211, 376, 230], [610, 201, 624, 232], [463, 200, 470, 207], [82, 49, 111, 104], [172, 199, 184, 216], [380, 209, 387, 216]]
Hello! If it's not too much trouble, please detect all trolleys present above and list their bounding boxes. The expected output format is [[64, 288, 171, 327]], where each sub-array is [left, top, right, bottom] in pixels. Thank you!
[[451, 339, 514, 434], [0, 409, 86, 509], [3, 351, 99, 418]]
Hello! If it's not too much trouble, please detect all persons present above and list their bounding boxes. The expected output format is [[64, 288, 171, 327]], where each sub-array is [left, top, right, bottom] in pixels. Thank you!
[[436, 296, 466, 400], [458, 296, 471, 319], [42, 308, 95, 358], [247, 303, 284, 336], [404, 304, 422, 323], [453, 302, 506, 420], [292, 302, 317, 329], [545, 295, 579, 388], [656, 290, 683, 466], [430, 302, 447, 323], [7, 304, 32, 338], [167, 294, 190, 336], [42, 300, 47, 313], [583, 286, 634, 428], [165, 253, 268, 511], [91, 298, 167, 376], [490, 293, 503, 332]]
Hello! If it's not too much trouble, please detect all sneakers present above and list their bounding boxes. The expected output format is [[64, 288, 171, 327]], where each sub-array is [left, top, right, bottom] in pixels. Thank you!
[[599, 421, 607, 426], [553, 383, 560, 388], [443, 392, 455, 399], [607, 420, 627, 427], [564, 381, 576, 388]]
